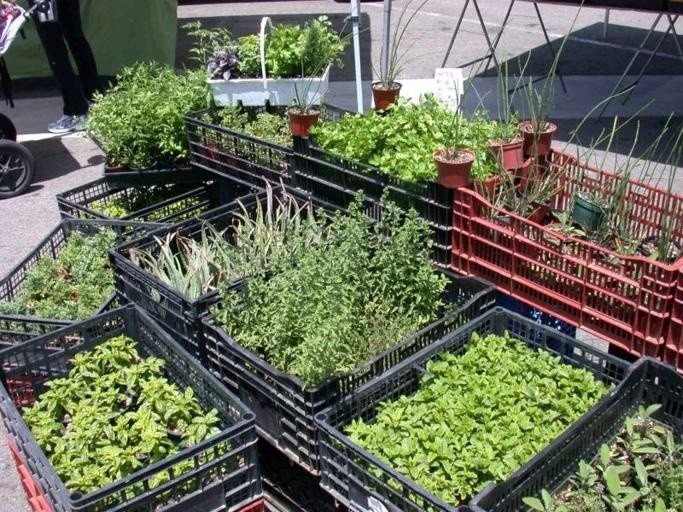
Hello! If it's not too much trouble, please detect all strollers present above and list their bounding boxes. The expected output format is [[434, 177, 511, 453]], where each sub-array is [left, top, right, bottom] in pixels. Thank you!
[[1, 0, 50, 197]]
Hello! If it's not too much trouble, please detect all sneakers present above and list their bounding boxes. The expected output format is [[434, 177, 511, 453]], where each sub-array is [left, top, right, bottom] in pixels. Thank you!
[[48, 115, 89, 134]]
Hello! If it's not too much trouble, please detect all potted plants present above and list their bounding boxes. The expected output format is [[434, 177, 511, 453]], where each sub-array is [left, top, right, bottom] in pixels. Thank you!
[[431, 63, 486, 189], [490, 59, 528, 173], [568, 127, 654, 228], [287, 21, 366, 141], [369, 2, 427, 113], [88, 61, 208, 171], [520, 0, 587, 161]]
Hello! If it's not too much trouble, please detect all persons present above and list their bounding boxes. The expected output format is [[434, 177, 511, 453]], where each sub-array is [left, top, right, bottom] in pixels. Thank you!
[[27, 0, 102, 133]]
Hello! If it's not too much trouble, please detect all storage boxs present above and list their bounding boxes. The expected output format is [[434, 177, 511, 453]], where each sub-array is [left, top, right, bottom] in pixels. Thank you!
[[203, 33, 332, 108], [280, 110, 524, 268], [317, 306, 634, 512], [51, 176, 220, 239], [199, 264, 495, 474], [184, 105, 362, 187], [0, 299, 263, 512], [105, 194, 348, 354], [0, 210, 169, 373], [470, 352, 683, 509], [453, 152, 683, 371]]
